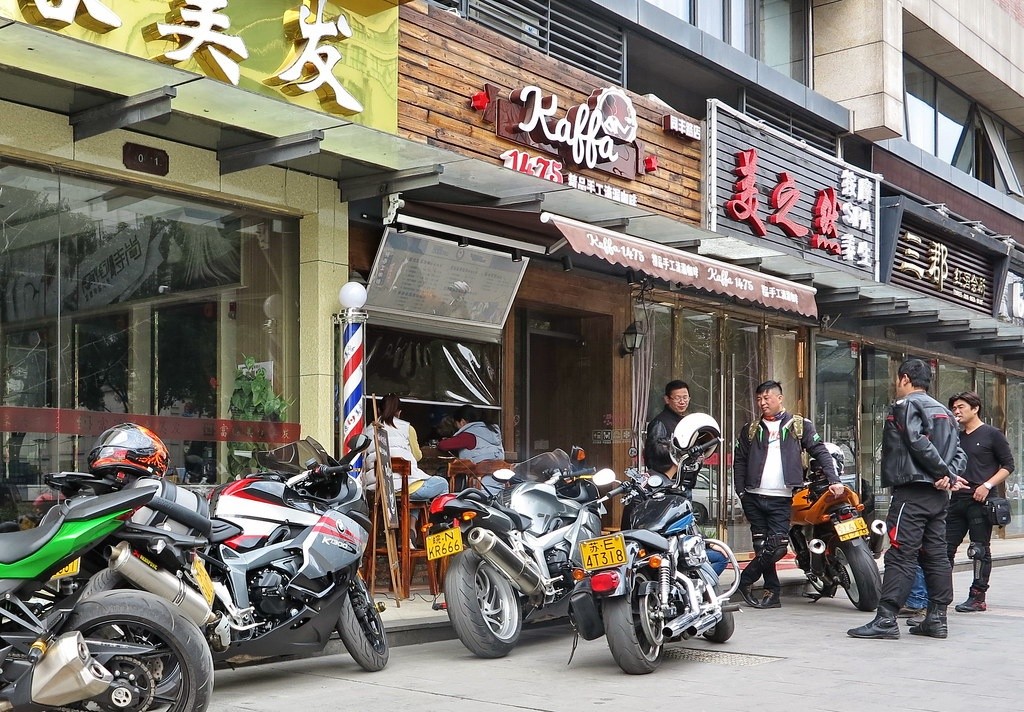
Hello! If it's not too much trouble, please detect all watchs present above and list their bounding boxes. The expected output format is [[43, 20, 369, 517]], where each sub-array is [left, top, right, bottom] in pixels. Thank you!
[[984, 481, 992, 489]]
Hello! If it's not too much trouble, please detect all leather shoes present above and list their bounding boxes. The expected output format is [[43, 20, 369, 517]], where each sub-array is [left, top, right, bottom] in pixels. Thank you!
[[731, 580, 759, 606], [754, 589, 780, 608]]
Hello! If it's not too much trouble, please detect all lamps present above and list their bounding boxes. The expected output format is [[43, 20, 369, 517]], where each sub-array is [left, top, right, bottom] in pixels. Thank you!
[[458, 236, 468, 247], [958, 221, 986, 235], [992, 235, 1017, 249], [619, 317, 646, 358], [263, 294, 283, 399], [511, 248, 522, 262], [330, 281, 368, 463], [561, 255, 573, 272], [626, 269, 635, 285], [397, 223, 407, 233], [923, 203, 949, 218]]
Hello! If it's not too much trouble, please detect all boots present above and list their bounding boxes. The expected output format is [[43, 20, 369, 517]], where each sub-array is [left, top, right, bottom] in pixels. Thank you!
[[909, 602, 947, 638], [846, 605, 900, 639], [955, 586, 986, 612]]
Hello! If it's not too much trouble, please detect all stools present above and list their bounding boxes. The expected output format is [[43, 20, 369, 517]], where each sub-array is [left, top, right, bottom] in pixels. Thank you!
[[365, 456, 512, 599]]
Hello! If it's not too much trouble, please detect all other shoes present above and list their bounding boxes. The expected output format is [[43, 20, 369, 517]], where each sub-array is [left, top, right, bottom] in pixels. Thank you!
[[906, 608, 928, 626], [408, 529, 418, 551], [898, 605, 918, 617]]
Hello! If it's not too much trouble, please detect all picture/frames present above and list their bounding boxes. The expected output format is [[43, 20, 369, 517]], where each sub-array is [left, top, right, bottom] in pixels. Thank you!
[[0, 206, 248, 326]]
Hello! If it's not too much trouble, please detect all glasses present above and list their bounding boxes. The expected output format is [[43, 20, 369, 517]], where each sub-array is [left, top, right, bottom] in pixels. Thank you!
[[668, 395, 691, 403]]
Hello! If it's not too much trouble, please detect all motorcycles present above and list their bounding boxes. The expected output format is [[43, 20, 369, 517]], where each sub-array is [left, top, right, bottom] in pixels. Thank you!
[[581, 437, 748, 676], [0, 482, 244, 712], [420, 444, 609, 659], [42, 435, 390, 673], [788, 465, 887, 612]]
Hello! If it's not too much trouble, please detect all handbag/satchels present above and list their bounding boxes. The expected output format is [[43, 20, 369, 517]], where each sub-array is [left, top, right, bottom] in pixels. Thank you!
[[982, 497, 1011, 526]]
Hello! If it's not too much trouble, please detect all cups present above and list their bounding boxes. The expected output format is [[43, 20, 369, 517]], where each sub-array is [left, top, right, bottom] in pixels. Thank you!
[[428, 439, 439, 448]]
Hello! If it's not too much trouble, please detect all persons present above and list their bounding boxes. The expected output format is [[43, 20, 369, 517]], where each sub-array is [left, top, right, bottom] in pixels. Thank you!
[[730, 380, 844, 609], [847, 358, 971, 639], [438, 404, 506, 500], [621, 438, 728, 577], [362, 392, 449, 550], [946, 391, 1015, 612], [643, 379, 703, 500]]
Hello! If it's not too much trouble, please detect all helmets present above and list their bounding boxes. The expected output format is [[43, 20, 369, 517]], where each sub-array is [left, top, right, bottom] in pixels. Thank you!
[[86, 423, 171, 480], [669, 413, 721, 472], [809, 442, 845, 477]]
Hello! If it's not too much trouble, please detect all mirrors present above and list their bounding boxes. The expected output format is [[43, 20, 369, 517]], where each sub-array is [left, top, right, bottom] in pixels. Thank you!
[[71, 308, 134, 473], [151, 293, 221, 486], [0, 322, 53, 498]]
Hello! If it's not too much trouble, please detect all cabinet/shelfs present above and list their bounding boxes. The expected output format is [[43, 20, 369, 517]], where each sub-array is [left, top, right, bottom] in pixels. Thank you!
[[823, 379, 888, 453]]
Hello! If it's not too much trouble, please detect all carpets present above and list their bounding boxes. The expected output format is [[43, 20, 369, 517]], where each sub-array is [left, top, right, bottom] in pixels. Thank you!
[[724, 563, 800, 570]]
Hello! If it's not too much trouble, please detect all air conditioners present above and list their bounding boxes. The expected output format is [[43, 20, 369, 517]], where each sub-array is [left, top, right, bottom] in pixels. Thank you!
[[468, 0, 540, 47]]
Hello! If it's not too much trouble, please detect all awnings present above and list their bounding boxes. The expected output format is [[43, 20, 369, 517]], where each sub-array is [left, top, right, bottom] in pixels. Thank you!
[[408, 197, 818, 321]]
